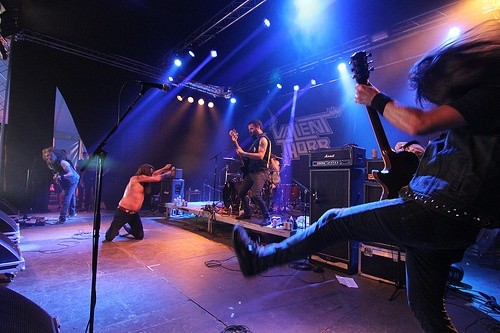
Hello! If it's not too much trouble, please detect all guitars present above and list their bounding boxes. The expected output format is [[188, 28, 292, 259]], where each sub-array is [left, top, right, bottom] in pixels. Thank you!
[[348, 50, 421, 202], [228, 129, 249, 176]]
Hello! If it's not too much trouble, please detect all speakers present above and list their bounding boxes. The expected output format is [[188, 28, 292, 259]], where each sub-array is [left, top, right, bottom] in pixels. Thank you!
[[0, 210, 18, 232], [308, 169, 366, 275], [0, 239, 20, 262], [0, 285, 60, 333], [161, 179, 185, 211]]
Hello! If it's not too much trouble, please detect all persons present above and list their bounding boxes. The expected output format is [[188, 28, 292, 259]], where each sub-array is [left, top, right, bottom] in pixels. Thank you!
[[235, 120, 271, 226], [266, 152, 279, 211], [105, 164, 175, 242], [42, 146, 80, 225], [233, 18, 500, 333]]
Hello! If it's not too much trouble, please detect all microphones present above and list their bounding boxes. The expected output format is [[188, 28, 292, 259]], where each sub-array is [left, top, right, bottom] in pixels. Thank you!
[[131, 80, 171, 93]]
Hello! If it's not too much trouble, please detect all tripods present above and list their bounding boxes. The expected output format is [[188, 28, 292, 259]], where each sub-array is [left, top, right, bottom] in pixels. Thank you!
[[202, 153, 222, 209]]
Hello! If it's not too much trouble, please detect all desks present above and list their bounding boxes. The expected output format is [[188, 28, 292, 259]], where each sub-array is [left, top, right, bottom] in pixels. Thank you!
[[186, 191, 201, 202]]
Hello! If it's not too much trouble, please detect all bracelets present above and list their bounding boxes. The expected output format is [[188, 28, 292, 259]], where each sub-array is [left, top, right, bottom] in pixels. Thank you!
[[371, 90, 395, 116]]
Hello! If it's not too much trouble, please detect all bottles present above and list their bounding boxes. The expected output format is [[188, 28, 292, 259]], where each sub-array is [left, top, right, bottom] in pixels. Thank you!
[[228, 205, 232, 214], [174, 195, 187, 207]]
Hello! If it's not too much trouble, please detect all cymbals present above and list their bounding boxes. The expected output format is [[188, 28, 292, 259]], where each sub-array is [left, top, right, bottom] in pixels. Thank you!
[[222, 158, 239, 162], [259, 169, 280, 173]]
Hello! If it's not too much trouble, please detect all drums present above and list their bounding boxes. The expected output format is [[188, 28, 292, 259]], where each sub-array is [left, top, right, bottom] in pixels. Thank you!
[[222, 179, 253, 211], [226, 160, 244, 177], [278, 183, 301, 206]]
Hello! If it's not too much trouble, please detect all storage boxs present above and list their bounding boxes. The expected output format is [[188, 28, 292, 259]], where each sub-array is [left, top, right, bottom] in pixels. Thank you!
[[358, 243, 406, 287]]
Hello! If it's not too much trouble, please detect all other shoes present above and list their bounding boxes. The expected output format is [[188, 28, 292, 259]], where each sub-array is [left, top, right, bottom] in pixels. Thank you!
[[68, 214, 77, 218], [233, 223, 268, 278], [55, 220, 65, 224], [236, 213, 251, 220], [260, 221, 271, 226]]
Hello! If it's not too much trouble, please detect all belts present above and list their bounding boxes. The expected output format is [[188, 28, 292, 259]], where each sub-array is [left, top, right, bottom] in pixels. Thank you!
[[118, 206, 139, 214]]
